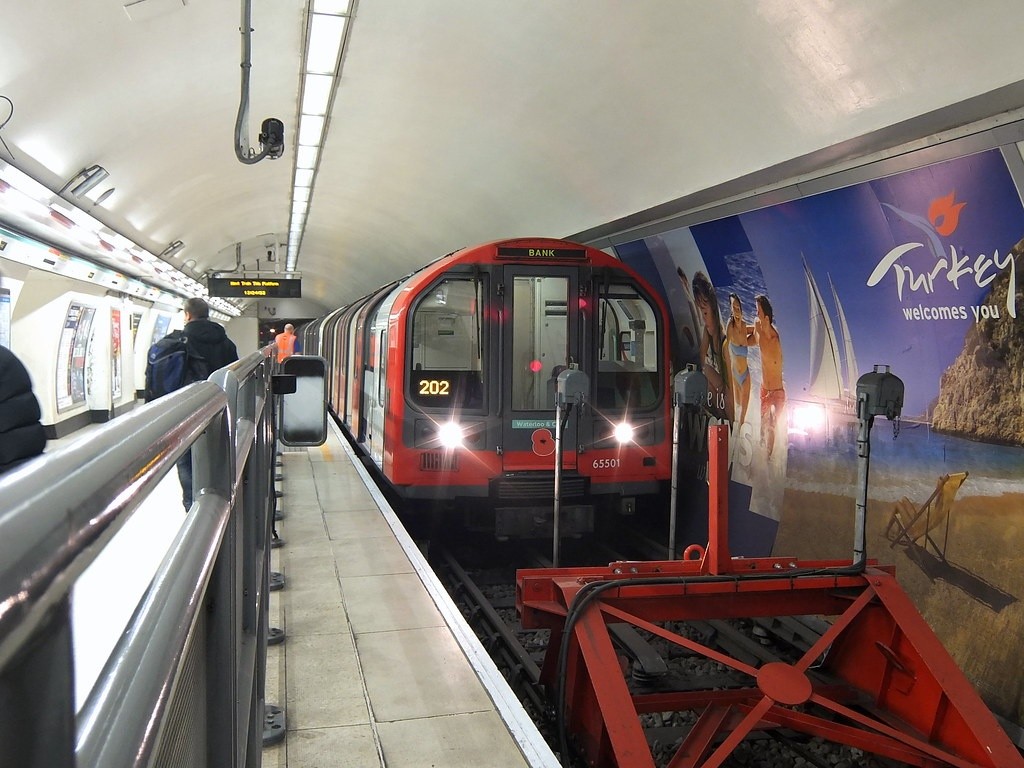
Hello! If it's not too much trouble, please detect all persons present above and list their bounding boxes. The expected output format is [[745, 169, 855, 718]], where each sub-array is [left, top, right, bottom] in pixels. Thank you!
[[146, 296, 239, 514], [274, 323, 303, 374], [681, 271, 787, 478], [0, 342, 79, 766]]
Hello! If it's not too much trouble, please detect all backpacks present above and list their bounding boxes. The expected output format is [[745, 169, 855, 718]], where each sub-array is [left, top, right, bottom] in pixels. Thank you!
[[144, 329, 209, 404]]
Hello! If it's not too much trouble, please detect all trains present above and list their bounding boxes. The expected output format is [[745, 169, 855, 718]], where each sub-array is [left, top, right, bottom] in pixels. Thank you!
[[294, 236, 673, 541]]
